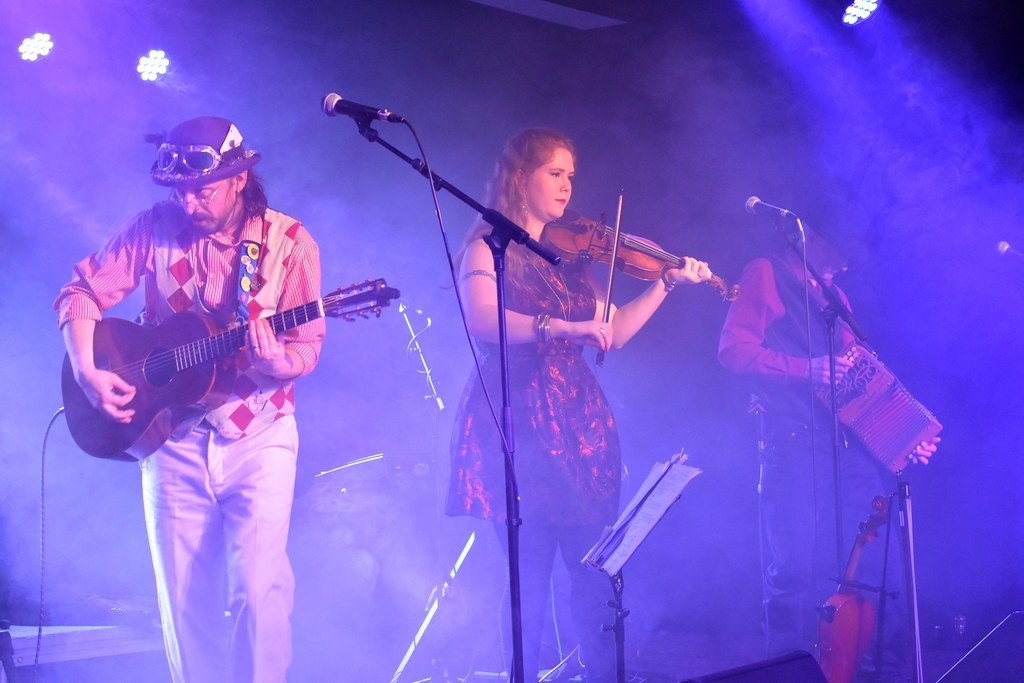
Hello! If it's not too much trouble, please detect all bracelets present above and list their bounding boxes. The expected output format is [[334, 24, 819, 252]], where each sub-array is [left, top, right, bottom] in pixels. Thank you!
[[535, 312, 555, 344], [666, 270, 678, 285]]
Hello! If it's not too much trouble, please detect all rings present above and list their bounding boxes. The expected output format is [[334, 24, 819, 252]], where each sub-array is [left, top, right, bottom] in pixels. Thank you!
[[600, 328, 606, 334], [251, 345, 260, 351], [697, 272, 704, 278]]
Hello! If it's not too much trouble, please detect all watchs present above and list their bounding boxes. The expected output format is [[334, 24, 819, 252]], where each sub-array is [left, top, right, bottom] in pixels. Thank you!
[[661, 273, 675, 291]]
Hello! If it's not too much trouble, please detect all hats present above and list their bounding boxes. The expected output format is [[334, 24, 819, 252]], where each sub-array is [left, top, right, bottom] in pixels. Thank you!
[[150, 116, 262, 186], [803, 191, 879, 266]]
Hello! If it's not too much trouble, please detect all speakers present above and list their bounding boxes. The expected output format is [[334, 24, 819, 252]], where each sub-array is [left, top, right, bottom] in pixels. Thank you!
[[675, 650, 829, 683]]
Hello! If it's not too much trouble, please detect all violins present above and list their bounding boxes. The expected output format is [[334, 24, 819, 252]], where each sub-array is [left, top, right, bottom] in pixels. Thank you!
[[815, 494, 890, 683], [543, 208, 741, 303]]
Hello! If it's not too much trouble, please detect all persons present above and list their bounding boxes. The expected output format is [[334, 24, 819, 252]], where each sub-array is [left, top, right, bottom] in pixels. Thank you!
[[717, 182, 940, 674], [445, 127, 712, 683], [54, 116, 326, 683]]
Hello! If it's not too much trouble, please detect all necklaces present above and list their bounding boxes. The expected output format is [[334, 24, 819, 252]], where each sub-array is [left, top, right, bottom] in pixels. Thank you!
[[527, 256, 570, 322]]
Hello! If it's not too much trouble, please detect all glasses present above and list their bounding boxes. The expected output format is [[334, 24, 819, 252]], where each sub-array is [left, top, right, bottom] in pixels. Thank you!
[[157, 142, 219, 174]]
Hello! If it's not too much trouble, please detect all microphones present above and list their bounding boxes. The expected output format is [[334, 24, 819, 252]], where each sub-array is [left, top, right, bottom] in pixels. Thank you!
[[994, 241, 1024, 260], [321, 92, 405, 122], [745, 195, 799, 220]]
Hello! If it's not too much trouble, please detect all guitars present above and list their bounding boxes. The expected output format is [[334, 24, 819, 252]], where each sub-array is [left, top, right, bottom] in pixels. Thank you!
[[61, 277, 400, 462]]
